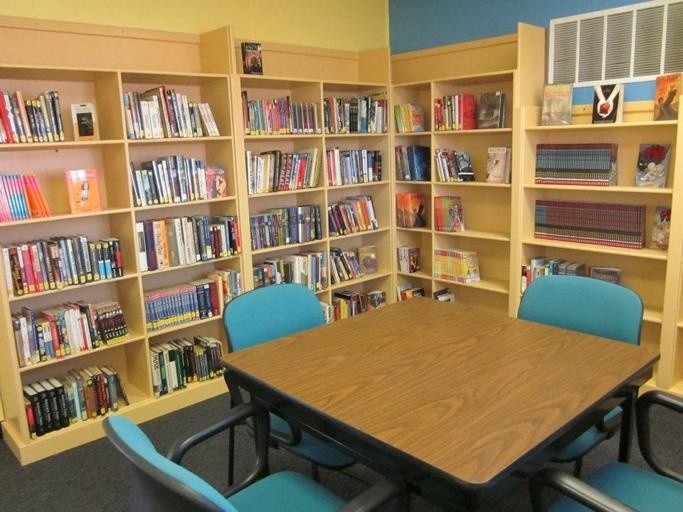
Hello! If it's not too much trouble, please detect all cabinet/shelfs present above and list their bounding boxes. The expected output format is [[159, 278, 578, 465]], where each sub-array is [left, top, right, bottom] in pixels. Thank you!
[[0, 13, 394, 470], [391, 22, 683, 399]]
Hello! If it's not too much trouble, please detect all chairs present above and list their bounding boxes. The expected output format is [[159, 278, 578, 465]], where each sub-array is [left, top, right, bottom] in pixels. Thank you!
[[514, 271, 645, 510], [101, 403, 411, 510], [533, 389, 681, 511]]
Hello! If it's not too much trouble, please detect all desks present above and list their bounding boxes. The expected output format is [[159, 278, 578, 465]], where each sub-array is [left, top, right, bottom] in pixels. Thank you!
[[217, 292, 663, 507]]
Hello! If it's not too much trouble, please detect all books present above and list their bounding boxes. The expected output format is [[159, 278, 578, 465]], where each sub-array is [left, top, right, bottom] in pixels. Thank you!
[[320, 290, 386, 325], [654, 74, 683, 122], [394, 102, 426, 134], [327, 148, 382, 185], [125, 86, 221, 140], [143, 268, 241, 333], [395, 191, 427, 228], [240, 42, 263, 75], [437, 146, 510, 184], [149, 335, 225, 398], [635, 144, 671, 188], [649, 206, 672, 253], [434, 248, 481, 284], [12, 300, 128, 367], [434, 196, 465, 232], [245, 149, 320, 194], [394, 146, 431, 182], [135, 214, 241, 271], [398, 246, 421, 273], [254, 250, 328, 292], [329, 247, 377, 284], [535, 198, 647, 250], [323, 94, 388, 135], [23, 362, 129, 436], [540, 83, 573, 128], [0, 173, 52, 222], [435, 92, 506, 130], [130, 154, 227, 206], [70, 104, 97, 142], [327, 195, 379, 237], [241, 90, 319, 135], [64, 168, 102, 214], [535, 144, 618, 187], [397, 283, 425, 304], [592, 85, 622, 123], [435, 288, 456, 304], [0, 90, 63, 144], [249, 205, 322, 252], [0, 233, 124, 297], [521, 256, 622, 300]]
